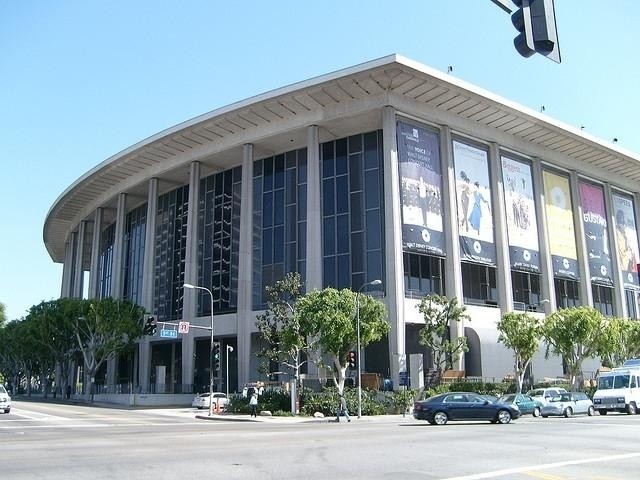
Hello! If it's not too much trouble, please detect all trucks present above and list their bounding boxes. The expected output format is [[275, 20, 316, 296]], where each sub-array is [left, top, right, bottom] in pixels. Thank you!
[[593, 361, 640, 416]]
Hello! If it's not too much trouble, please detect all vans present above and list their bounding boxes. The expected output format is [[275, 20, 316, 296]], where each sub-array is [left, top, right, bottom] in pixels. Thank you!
[[526, 387, 567, 406]]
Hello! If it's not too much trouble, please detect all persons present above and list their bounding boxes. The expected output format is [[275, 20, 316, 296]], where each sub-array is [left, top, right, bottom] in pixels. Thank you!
[[52, 384, 57, 398], [458, 172, 472, 231], [335, 392, 351, 422], [383, 376, 392, 391], [468, 181, 489, 235], [507, 174, 529, 229], [615, 210, 634, 271], [249, 393, 258, 417], [67, 384, 71, 399], [417, 175, 428, 227]]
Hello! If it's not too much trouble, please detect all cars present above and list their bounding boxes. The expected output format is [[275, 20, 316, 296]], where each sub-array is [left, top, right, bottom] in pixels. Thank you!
[[493, 393, 543, 417], [413, 392, 521, 424], [542, 391, 594, 418], [0, 384, 12, 414], [192, 391, 232, 410]]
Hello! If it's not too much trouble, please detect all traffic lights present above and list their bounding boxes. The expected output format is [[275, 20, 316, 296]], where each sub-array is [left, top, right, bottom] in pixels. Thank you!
[[144, 317, 158, 337], [511, 0, 561, 65], [348, 350, 356, 370], [212, 341, 221, 374]]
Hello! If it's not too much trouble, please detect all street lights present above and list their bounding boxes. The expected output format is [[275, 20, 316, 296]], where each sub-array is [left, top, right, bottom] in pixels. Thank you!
[[356, 279, 382, 417], [226, 344, 234, 404], [183, 284, 214, 417]]
